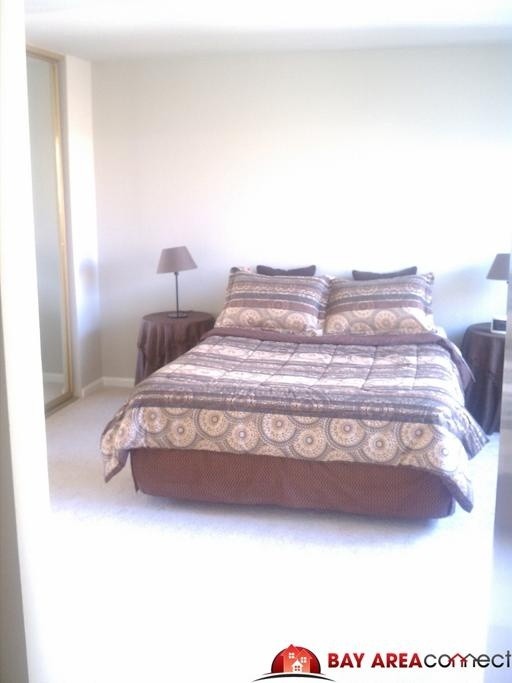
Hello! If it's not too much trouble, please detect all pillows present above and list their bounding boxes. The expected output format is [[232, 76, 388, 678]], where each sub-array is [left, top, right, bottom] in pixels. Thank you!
[[213, 265, 437, 335]]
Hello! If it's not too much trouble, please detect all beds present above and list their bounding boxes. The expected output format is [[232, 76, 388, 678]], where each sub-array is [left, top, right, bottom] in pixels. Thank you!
[[125, 336, 464, 518]]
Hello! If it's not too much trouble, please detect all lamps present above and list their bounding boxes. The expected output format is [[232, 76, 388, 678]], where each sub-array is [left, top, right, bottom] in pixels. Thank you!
[[487, 251, 511, 285], [156, 245, 199, 319]]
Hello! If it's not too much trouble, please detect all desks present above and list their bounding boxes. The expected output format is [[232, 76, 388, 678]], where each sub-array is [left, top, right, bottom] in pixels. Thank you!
[[138, 312, 215, 381], [460, 322, 505, 434]]
